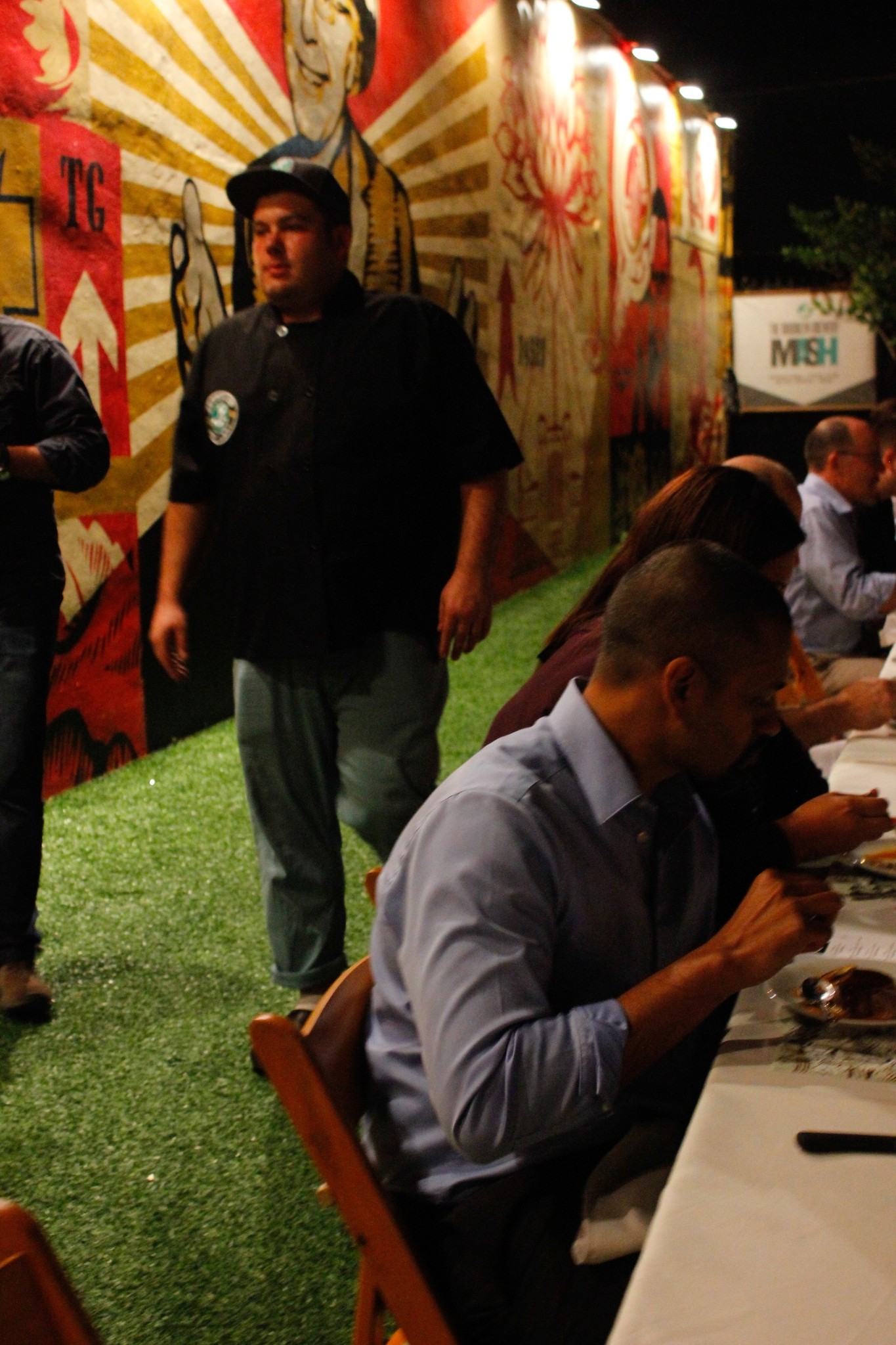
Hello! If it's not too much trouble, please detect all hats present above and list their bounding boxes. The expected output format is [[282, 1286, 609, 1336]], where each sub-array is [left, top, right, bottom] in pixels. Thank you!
[[225, 163, 353, 229]]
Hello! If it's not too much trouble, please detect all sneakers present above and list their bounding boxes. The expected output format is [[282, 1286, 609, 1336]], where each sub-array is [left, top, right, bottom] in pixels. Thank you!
[[0, 960, 53, 1014]]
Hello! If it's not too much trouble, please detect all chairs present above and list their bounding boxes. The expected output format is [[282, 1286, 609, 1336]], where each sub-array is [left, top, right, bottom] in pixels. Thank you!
[[248, 867, 459, 1345]]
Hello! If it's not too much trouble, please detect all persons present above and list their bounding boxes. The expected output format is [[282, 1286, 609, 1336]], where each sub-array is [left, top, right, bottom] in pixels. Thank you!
[[358, 540, 848, 1344], [718, 455, 895, 757], [145, 157, 527, 1078], [0, 311, 113, 1023], [780, 415, 896, 698], [479, 460, 896, 923]]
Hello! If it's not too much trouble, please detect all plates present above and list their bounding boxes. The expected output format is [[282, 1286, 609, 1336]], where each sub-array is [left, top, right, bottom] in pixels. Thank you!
[[772, 955, 896, 1033], [834, 837, 896, 883]]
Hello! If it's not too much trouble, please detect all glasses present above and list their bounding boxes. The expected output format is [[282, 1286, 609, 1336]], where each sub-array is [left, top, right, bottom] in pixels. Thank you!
[[828, 450, 881, 462]]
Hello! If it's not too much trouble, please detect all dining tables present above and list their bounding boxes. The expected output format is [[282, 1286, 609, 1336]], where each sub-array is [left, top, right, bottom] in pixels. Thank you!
[[604, 725, 896, 1344]]
